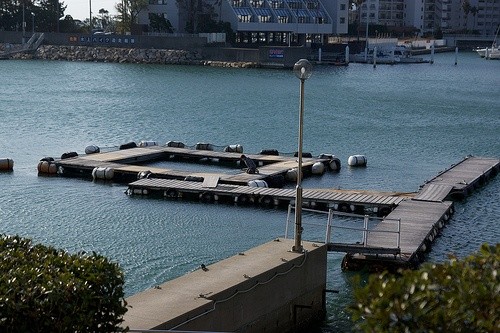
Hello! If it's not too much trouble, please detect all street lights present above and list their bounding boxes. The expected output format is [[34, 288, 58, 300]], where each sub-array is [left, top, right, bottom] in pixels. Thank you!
[[293, 58, 313, 252]]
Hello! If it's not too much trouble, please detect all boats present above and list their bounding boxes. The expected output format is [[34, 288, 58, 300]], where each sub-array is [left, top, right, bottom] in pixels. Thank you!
[[476, 25, 500, 59]]
